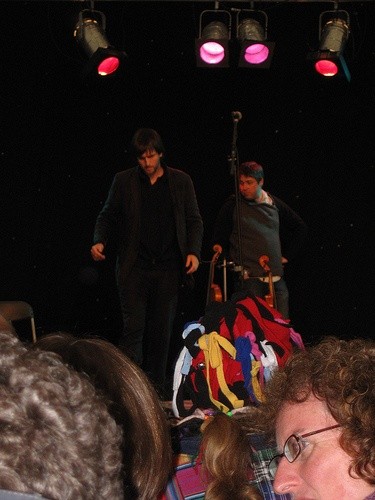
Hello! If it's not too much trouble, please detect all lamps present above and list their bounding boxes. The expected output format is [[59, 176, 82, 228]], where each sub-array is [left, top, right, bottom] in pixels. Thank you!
[[231, 0, 270, 67], [194, 0, 233, 65], [314, 0, 352, 76], [71, 0, 126, 78]]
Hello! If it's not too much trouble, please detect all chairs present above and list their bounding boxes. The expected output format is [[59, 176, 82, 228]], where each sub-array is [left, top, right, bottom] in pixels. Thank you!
[[0, 298, 37, 346]]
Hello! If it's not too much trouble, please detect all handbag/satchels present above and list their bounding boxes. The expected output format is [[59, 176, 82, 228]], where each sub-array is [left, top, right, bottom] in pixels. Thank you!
[[172, 294, 305, 419]]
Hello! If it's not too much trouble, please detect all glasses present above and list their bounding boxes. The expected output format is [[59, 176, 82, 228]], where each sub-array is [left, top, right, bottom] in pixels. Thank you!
[[268, 422, 350, 479]]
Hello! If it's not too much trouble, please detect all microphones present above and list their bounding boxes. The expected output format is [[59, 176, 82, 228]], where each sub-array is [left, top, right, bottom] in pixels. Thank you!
[[232, 110, 243, 119]]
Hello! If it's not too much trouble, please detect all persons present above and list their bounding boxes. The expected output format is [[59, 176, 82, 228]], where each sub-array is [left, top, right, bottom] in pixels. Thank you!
[[213, 161, 307, 317], [0, 291, 307, 500], [91, 128, 203, 400], [254, 336, 375, 500]]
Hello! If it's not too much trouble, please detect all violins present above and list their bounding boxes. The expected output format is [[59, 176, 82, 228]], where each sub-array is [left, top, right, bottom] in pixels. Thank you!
[[204, 244, 224, 314], [259, 256, 278, 310]]
